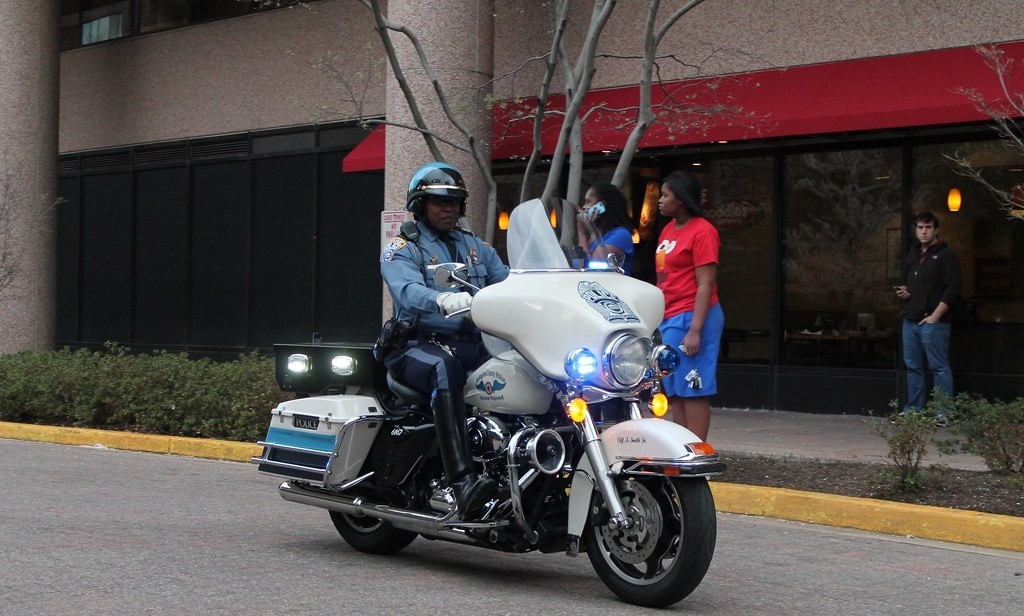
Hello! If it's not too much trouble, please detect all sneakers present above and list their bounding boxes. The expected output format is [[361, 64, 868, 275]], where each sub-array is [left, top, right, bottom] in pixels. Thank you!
[[890, 411, 906, 425], [936, 414, 960, 427]]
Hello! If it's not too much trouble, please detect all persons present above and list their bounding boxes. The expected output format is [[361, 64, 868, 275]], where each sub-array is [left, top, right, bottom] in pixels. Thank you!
[[655, 172, 725, 442], [577, 182, 634, 276], [889, 212, 961, 426], [381, 162, 509, 522]]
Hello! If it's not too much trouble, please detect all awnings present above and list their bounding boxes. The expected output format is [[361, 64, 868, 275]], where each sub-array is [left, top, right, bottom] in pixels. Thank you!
[[342, 39, 1024, 172]]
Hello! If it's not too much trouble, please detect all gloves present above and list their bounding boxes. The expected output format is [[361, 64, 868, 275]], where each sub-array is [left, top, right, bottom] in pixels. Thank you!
[[436, 291, 473, 319]]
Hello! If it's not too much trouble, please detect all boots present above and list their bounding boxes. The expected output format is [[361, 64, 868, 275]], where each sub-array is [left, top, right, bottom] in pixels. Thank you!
[[430, 388, 498, 522]]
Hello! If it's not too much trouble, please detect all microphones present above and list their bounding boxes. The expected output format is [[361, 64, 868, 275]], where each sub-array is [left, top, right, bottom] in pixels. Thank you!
[[451, 215, 465, 219]]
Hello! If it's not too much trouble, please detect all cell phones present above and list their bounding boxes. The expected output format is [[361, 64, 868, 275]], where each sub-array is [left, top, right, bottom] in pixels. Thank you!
[[893, 287, 902, 291], [584, 202, 606, 218]]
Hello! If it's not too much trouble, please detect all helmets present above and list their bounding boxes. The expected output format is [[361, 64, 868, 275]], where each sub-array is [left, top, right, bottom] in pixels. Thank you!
[[406, 162, 471, 218]]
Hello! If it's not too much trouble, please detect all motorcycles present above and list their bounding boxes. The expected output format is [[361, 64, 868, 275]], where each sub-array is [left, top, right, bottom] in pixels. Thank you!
[[249, 196, 728, 607]]
